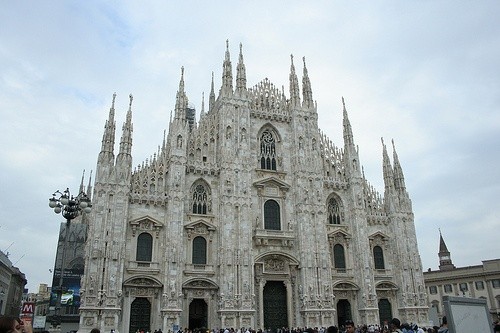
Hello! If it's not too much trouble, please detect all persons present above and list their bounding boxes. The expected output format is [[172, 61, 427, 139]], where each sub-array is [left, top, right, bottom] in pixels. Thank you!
[[89, 315, 452, 333]]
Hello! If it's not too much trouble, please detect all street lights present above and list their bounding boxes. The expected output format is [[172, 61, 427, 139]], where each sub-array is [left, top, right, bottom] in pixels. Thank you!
[[52, 187, 92, 332]]
